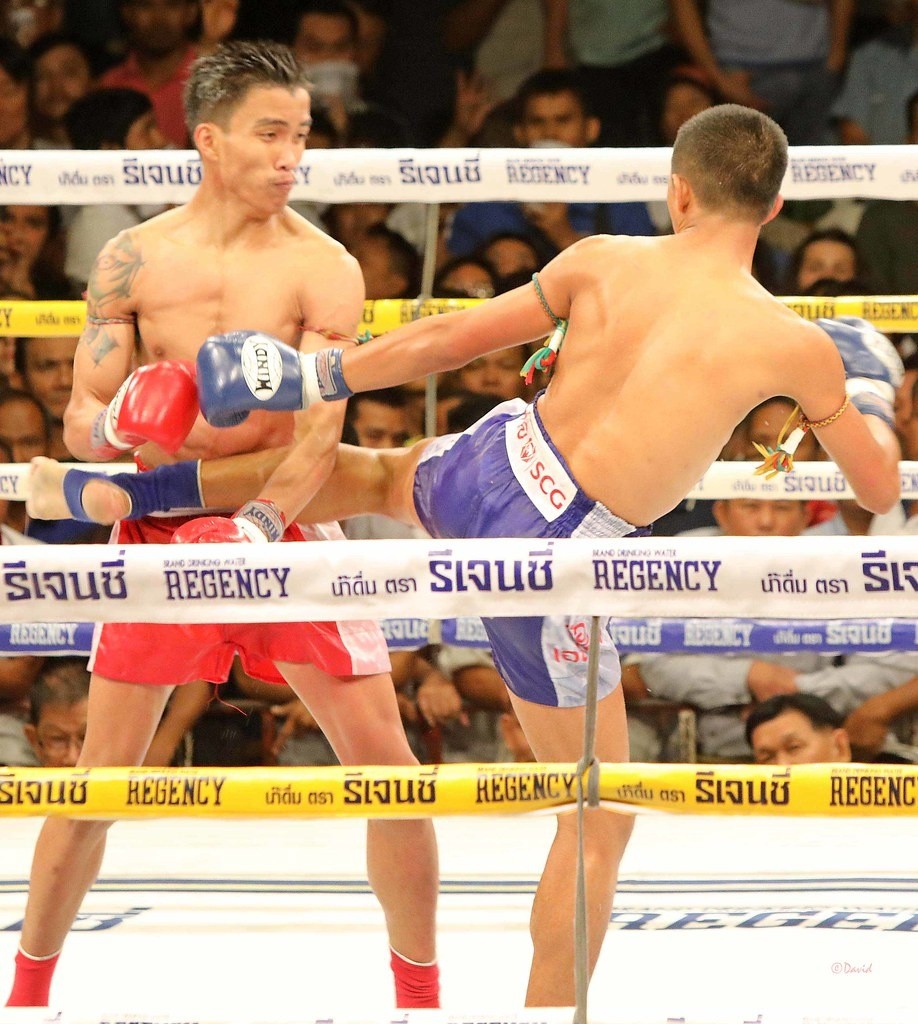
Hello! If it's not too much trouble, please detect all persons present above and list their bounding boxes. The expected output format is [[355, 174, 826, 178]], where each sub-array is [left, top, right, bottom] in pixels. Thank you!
[[22, 101, 904, 1006], [1, 0, 918, 770], [6, 43, 447, 1007]]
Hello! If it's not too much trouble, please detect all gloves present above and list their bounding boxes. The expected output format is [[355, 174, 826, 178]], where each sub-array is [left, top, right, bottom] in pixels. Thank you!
[[168, 498, 286, 543], [817, 316, 904, 424], [104, 359, 201, 456], [195, 324, 353, 433]]
